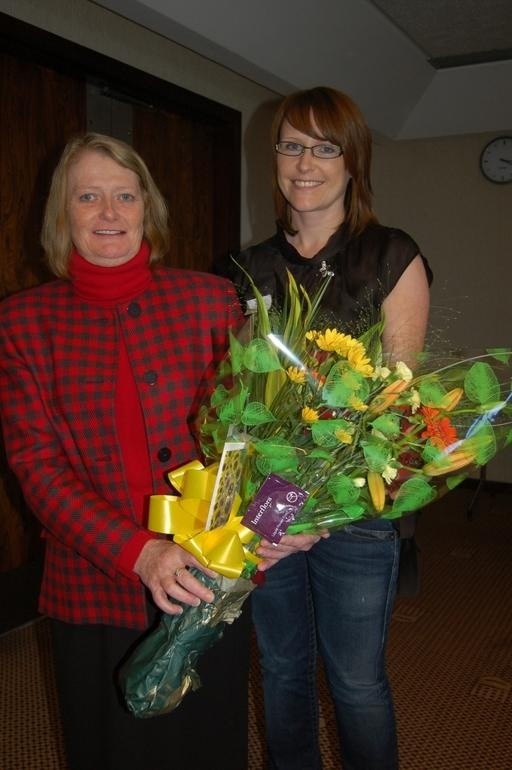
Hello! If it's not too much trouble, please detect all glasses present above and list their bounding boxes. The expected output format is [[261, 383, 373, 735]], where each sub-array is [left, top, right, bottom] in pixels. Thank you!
[[273, 138, 346, 161]]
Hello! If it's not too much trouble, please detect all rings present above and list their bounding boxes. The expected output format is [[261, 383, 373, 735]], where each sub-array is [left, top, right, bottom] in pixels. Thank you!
[[175, 567, 187, 578]]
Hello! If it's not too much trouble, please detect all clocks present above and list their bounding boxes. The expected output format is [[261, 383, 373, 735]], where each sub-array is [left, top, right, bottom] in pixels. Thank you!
[[479, 136, 512, 184]]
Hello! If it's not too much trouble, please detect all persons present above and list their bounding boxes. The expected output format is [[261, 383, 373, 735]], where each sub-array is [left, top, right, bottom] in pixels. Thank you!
[[218, 85, 434, 770], [1, 127, 331, 769]]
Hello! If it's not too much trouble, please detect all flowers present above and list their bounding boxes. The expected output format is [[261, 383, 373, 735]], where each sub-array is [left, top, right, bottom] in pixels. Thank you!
[[116, 248, 512, 717]]
